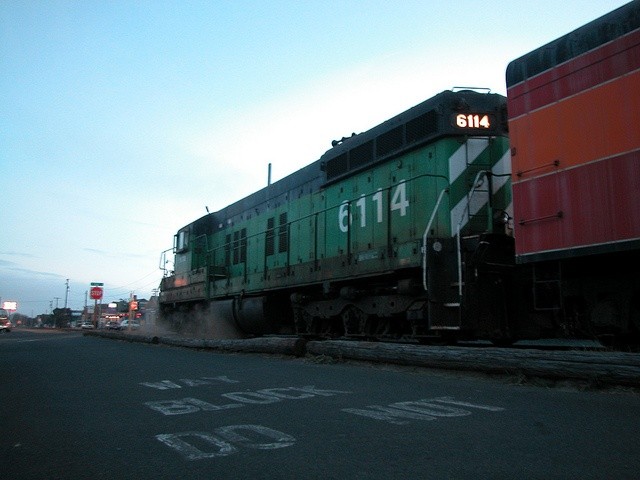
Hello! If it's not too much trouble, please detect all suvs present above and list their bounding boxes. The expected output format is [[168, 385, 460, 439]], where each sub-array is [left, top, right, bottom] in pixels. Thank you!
[[0, 314, 12, 332]]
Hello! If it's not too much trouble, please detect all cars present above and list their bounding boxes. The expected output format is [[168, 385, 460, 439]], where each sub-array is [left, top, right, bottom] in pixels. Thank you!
[[104, 321, 121, 330], [81, 322, 94, 329]]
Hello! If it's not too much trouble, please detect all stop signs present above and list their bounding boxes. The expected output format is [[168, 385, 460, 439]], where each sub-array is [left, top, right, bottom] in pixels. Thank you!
[[131, 302, 138, 310], [90, 287, 103, 300]]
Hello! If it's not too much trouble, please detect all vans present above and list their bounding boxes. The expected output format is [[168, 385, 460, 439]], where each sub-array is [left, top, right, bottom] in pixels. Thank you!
[[121, 320, 139, 327]]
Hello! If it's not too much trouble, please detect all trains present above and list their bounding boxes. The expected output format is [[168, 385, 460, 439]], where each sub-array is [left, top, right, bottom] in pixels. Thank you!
[[153, 0, 640, 351]]
